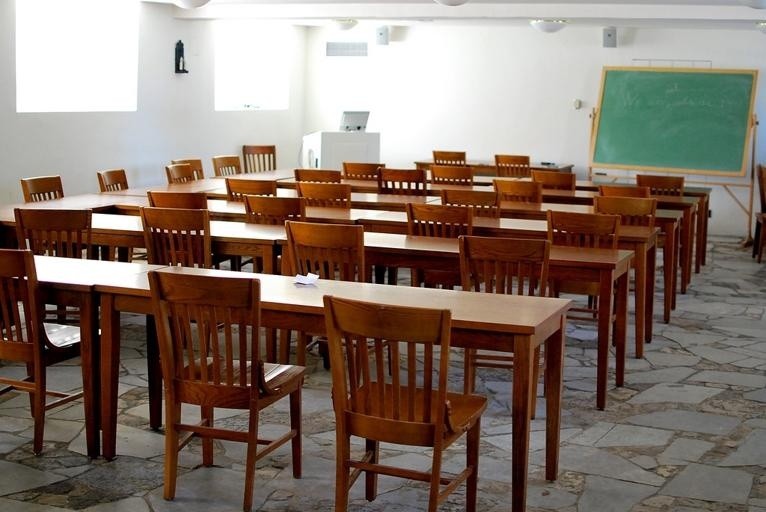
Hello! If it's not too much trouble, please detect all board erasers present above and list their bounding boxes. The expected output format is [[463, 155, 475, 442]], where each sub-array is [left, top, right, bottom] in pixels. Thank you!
[[595, 172, 606, 175]]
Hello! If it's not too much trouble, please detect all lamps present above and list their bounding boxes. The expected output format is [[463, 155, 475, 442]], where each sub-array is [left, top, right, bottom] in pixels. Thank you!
[[169, 0, 208, 9], [434, 0, 467, 7], [325, 18, 358, 33], [528, 20, 570, 35]]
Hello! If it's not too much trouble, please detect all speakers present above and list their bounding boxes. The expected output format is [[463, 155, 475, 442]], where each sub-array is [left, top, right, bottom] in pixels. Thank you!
[[325, 41, 369, 57]]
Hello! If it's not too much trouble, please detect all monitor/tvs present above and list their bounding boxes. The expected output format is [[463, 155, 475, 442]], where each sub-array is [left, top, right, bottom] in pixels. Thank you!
[[338, 110, 370, 131]]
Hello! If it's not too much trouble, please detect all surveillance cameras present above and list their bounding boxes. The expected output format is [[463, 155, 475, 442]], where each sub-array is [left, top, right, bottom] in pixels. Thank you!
[[369, 18, 391, 46]]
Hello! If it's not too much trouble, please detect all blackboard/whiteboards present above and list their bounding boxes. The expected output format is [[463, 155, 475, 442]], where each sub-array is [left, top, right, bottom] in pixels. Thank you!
[[589, 66, 758, 177]]
